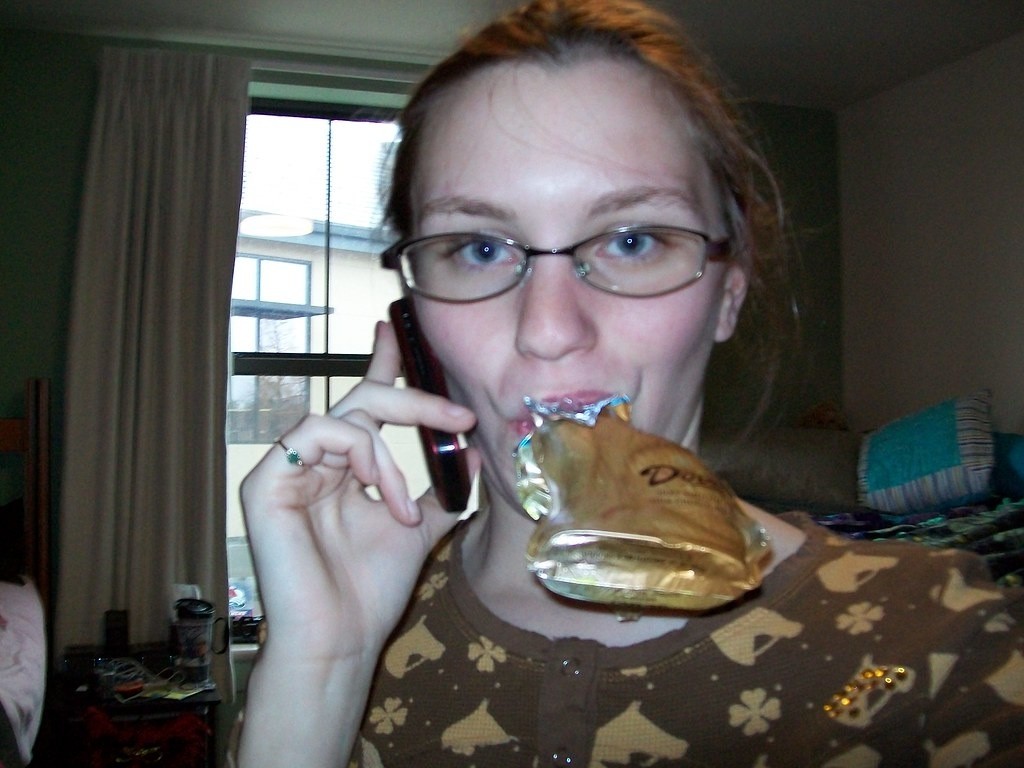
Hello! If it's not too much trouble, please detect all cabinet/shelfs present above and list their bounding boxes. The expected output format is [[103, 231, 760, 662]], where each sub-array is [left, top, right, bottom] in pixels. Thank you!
[[61, 645, 223, 768]]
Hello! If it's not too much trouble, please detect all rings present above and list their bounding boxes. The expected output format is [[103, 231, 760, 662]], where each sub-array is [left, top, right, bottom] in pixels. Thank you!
[[274, 440, 303, 465]]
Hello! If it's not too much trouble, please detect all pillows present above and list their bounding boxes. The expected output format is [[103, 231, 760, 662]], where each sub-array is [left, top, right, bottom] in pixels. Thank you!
[[991, 432, 1024, 499], [856, 388, 1001, 510], [697, 423, 861, 512]]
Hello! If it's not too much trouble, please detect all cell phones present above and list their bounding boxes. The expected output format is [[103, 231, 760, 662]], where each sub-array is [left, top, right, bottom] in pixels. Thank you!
[[389, 298, 471, 514]]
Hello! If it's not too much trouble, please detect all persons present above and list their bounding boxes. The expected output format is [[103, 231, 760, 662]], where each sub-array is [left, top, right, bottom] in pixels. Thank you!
[[238, 1, 1024, 768]]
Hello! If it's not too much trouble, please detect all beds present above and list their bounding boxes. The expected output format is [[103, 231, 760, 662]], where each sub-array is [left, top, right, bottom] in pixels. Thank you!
[[735, 493, 1024, 602]]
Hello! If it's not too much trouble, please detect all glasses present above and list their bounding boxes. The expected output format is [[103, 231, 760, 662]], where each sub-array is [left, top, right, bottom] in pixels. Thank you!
[[381, 224, 731, 304]]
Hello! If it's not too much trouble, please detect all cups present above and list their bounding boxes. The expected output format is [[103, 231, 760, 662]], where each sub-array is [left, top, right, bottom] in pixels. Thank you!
[[174, 598, 216, 680]]
